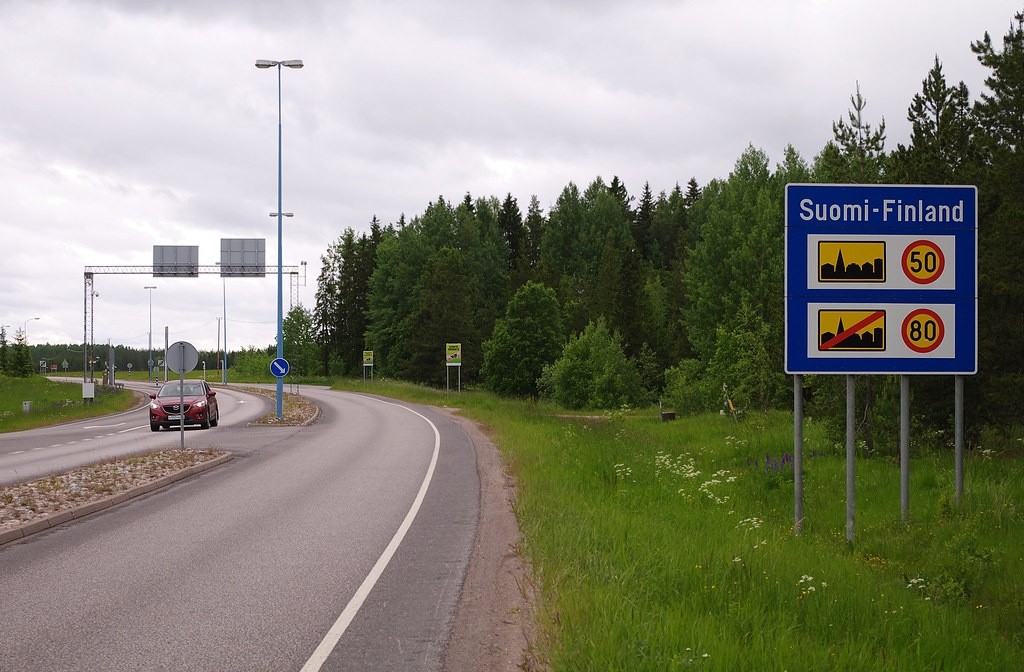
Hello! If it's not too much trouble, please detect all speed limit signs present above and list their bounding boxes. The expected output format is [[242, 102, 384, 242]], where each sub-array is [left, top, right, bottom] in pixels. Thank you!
[[784, 183, 978, 374]]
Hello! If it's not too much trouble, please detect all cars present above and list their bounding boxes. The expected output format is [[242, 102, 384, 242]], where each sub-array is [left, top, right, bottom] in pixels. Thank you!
[[149, 379, 220, 432]]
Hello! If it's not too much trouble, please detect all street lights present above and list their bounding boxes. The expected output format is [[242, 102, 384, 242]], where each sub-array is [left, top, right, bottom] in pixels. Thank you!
[[254, 57, 304, 418], [24, 318, 40, 345], [214, 261, 228, 386], [144, 285, 157, 383]]
